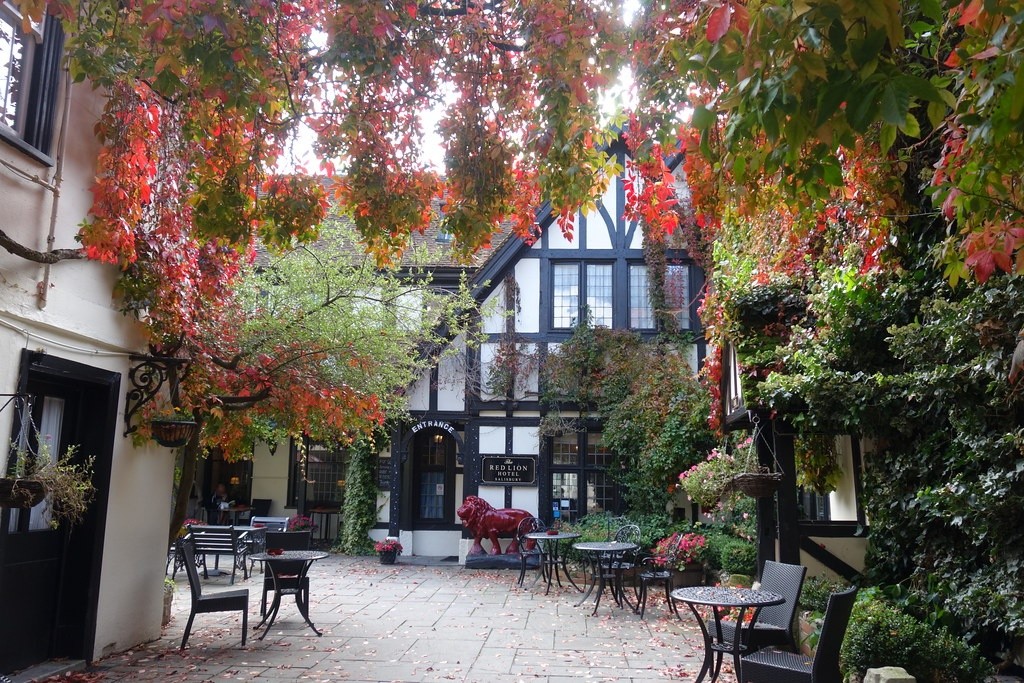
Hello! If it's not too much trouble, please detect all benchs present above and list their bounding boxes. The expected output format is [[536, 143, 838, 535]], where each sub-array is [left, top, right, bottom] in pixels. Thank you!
[[251, 516, 290, 551], [185, 524, 248, 580]]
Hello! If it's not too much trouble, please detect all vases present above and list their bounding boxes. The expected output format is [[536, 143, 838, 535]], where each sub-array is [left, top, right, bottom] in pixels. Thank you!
[[151, 419, 195, 448], [378, 550, 397, 565], [721, 469, 782, 510]]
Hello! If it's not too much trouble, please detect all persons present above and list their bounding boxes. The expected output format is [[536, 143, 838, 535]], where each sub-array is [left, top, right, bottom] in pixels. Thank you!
[[206, 482, 234, 509]]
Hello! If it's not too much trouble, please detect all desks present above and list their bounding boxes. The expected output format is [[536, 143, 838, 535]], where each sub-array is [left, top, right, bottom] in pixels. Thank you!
[[572, 543, 640, 618], [669, 587, 786, 683], [312, 509, 339, 544], [251, 551, 331, 639], [206, 505, 249, 525], [204, 525, 267, 576], [525, 533, 580, 597]]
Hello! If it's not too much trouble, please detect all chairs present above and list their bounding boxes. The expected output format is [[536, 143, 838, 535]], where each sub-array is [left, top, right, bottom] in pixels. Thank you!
[[261, 531, 312, 619], [638, 531, 684, 626], [248, 499, 272, 523], [516, 516, 552, 587], [595, 524, 641, 612], [181, 532, 248, 649], [740, 586, 856, 683], [710, 560, 808, 677]]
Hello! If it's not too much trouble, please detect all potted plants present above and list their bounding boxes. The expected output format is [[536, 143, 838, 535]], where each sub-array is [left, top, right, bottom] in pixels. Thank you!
[[0, 446, 100, 532]]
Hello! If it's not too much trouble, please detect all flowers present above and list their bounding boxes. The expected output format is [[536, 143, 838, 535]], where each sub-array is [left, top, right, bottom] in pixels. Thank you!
[[135, 398, 196, 448], [373, 541, 404, 554], [678, 437, 754, 520], [290, 515, 318, 532]]
